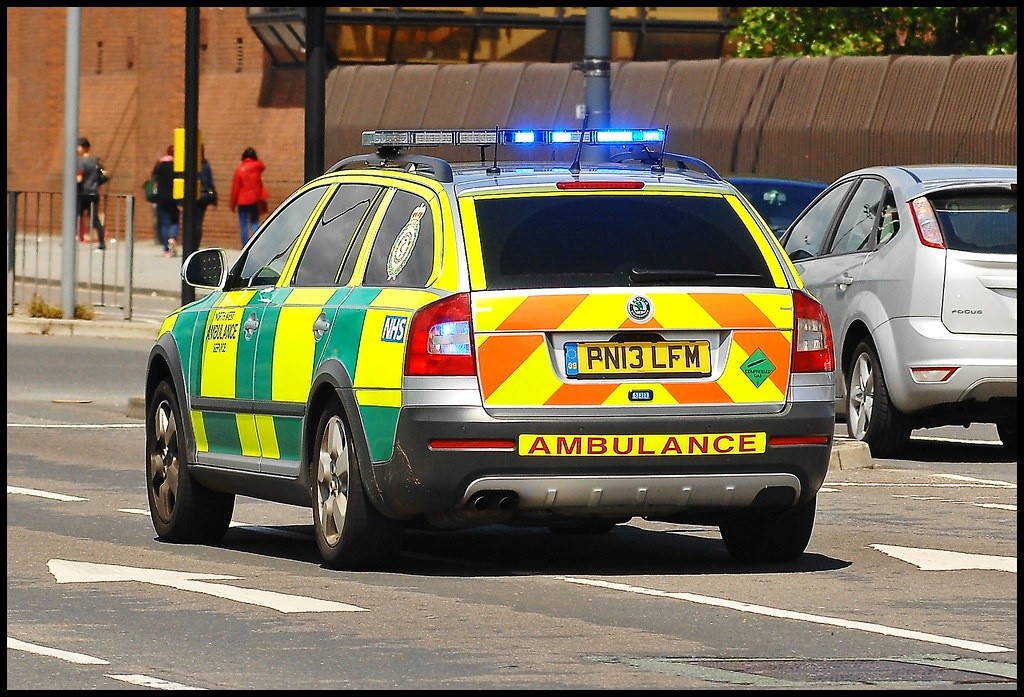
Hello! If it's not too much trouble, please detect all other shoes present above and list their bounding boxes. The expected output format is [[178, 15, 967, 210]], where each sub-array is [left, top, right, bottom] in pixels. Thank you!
[[95, 246, 106, 253], [164, 238, 177, 257]]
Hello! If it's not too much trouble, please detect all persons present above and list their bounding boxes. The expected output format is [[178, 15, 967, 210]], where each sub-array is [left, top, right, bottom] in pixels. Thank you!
[[194, 143, 218, 251], [229, 147, 270, 245], [76, 137, 111, 250], [152, 144, 180, 258]]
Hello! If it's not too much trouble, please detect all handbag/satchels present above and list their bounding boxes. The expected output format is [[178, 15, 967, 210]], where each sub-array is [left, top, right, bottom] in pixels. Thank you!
[[96, 157, 109, 186], [143, 177, 158, 203], [208, 186, 216, 206], [258, 201, 268, 214]]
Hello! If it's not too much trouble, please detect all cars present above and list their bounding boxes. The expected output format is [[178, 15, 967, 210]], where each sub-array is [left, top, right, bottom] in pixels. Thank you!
[[143, 124, 840, 566], [722, 174, 829, 246], [770, 163, 1021, 458]]
